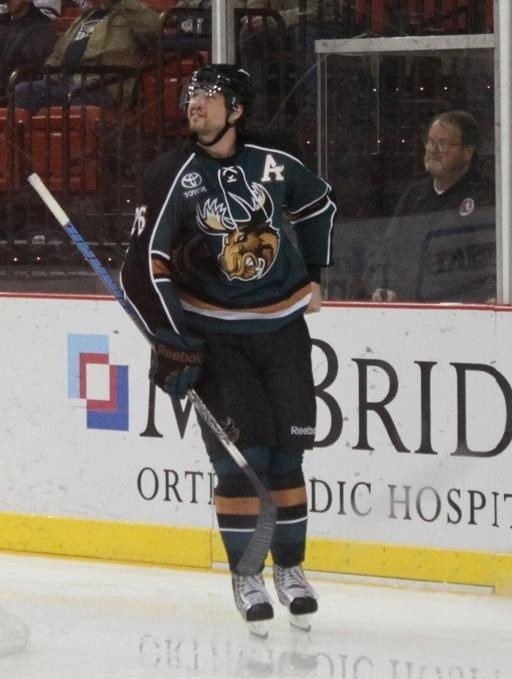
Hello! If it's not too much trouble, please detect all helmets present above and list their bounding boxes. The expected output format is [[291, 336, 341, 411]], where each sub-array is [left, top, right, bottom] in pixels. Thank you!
[[179, 63, 256, 129]]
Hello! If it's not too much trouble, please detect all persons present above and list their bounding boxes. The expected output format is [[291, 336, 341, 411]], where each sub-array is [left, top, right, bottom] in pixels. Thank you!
[[370, 109, 497, 307], [117, 63, 340, 623]]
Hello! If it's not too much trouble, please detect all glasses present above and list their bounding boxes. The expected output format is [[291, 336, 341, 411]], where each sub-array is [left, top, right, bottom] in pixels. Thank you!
[[421, 136, 462, 154]]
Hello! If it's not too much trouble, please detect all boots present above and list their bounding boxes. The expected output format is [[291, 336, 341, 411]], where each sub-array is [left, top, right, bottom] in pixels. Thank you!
[[272, 562, 319, 615], [231, 573, 274, 622]]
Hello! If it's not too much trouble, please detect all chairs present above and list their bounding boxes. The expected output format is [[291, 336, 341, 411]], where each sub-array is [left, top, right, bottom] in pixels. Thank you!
[[1, 34, 206, 216]]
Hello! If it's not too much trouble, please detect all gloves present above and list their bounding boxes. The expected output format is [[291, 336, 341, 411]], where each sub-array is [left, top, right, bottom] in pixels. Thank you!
[[148, 336, 208, 401]]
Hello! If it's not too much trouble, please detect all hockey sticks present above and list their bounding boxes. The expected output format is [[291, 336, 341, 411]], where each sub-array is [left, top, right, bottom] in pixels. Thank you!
[[28, 173, 276, 576]]
